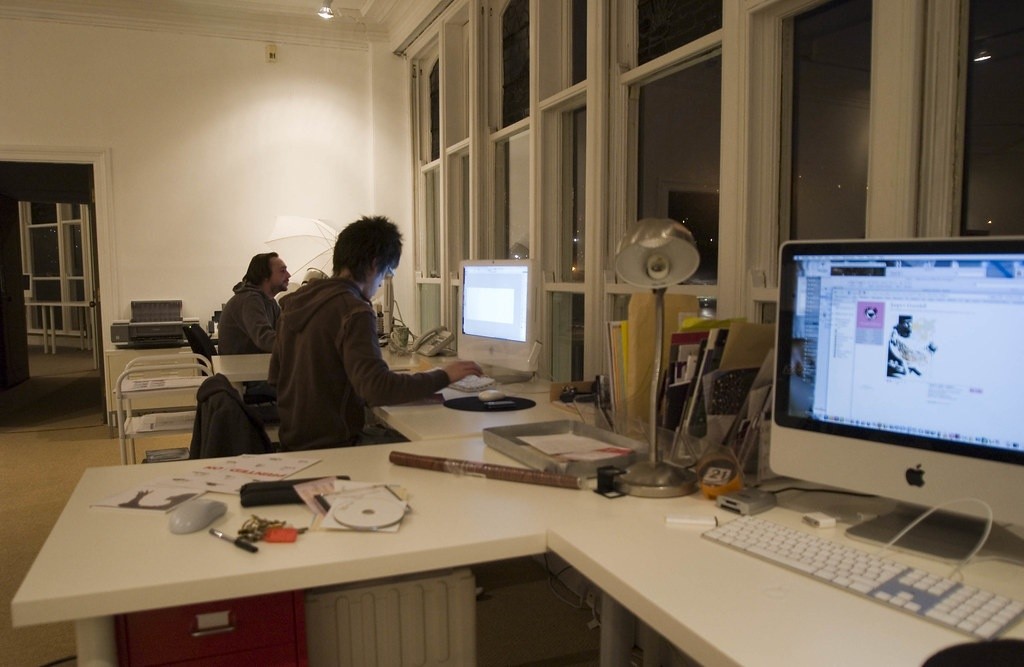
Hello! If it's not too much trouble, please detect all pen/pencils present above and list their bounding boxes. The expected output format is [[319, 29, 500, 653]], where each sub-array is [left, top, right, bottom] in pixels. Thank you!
[[209, 527, 258, 553]]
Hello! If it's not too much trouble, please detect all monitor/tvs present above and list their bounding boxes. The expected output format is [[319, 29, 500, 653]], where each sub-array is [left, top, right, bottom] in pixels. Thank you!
[[381, 269, 394, 333], [769, 234, 1023, 567], [458, 259, 543, 383]]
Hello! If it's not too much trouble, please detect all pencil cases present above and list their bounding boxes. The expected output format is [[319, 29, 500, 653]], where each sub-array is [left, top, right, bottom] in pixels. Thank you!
[[240, 475, 350, 508]]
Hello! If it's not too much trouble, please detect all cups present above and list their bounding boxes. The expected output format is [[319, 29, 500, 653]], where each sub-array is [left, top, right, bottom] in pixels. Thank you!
[[391, 326, 410, 347]]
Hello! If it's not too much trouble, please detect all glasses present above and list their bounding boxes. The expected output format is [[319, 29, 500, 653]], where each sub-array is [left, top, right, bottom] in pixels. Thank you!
[[384, 264, 396, 281]]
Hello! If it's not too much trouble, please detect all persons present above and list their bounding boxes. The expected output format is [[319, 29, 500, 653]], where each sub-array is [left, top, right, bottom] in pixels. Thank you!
[[886, 313, 926, 378], [276, 217, 484, 450], [217, 253, 291, 407]]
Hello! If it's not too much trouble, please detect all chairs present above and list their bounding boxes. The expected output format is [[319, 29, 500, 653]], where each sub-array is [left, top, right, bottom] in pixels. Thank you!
[[186, 372, 284, 460], [184, 325, 219, 376]]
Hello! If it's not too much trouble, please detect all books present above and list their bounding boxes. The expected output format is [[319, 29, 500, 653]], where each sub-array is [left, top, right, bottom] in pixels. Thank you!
[[88, 453, 323, 514], [604, 289, 776, 472]]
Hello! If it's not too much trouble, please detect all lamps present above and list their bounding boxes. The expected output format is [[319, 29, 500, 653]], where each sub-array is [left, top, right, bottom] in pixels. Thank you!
[[614, 215, 699, 499], [317, 0, 335, 19]]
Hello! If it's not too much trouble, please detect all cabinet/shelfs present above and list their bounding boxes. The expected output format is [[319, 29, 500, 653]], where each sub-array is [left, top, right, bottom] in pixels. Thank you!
[[115, 587, 308, 667]]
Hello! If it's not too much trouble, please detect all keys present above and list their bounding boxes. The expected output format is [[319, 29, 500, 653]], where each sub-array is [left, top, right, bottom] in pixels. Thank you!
[[237, 514, 307, 543]]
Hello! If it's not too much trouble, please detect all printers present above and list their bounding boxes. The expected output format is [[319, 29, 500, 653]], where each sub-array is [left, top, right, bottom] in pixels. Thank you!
[[110, 300, 200, 348]]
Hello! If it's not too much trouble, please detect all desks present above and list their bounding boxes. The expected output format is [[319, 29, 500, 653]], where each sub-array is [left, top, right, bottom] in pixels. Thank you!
[[8, 337, 1024, 667]]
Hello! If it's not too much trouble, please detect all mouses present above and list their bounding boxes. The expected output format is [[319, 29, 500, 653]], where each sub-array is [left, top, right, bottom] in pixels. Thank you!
[[478, 390, 505, 402], [167, 498, 227, 535]]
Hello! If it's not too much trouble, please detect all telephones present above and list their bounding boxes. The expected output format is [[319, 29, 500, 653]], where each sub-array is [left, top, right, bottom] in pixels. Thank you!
[[412, 326, 454, 356]]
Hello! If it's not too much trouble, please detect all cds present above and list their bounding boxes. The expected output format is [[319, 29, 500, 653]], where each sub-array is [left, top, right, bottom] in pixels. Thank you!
[[333, 499, 405, 528]]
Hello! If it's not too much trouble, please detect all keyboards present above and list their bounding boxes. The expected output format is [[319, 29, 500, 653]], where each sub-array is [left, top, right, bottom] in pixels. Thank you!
[[700, 513, 1024, 642], [425, 367, 496, 392]]
